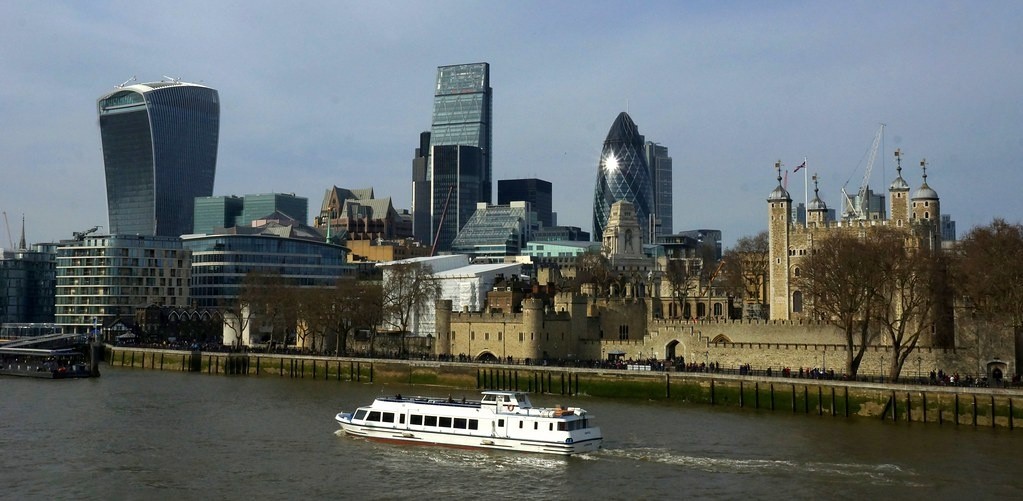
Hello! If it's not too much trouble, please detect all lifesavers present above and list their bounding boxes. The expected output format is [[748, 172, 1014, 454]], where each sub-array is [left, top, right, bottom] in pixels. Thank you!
[[508, 405, 514, 411]]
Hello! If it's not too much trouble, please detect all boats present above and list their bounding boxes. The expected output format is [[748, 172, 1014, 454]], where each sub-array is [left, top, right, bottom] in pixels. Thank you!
[[0, 340, 101, 379], [334, 391, 603, 456]]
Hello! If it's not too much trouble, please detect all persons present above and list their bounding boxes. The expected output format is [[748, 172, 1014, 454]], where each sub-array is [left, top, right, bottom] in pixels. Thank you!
[[843, 374, 848, 380], [486, 356, 489, 361], [966, 373, 971, 380], [929, 369, 959, 386], [461, 395, 466, 404], [395, 393, 402, 400], [507, 354, 513, 363], [558, 358, 565, 367], [285, 346, 337, 356], [782, 366, 834, 380], [766, 366, 772, 376], [447, 394, 452, 403], [140, 340, 230, 353], [390, 350, 397, 358], [975, 378, 980, 385], [596, 359, 664, 371], [499, 356, 501, 363], [672, 355, 719, 374], [345, 347, 369, 358], [574, 359, 580, 367], [743, 363, 750, 374], [247, 345, 282, 353], [420, 352, 471, 363]]
